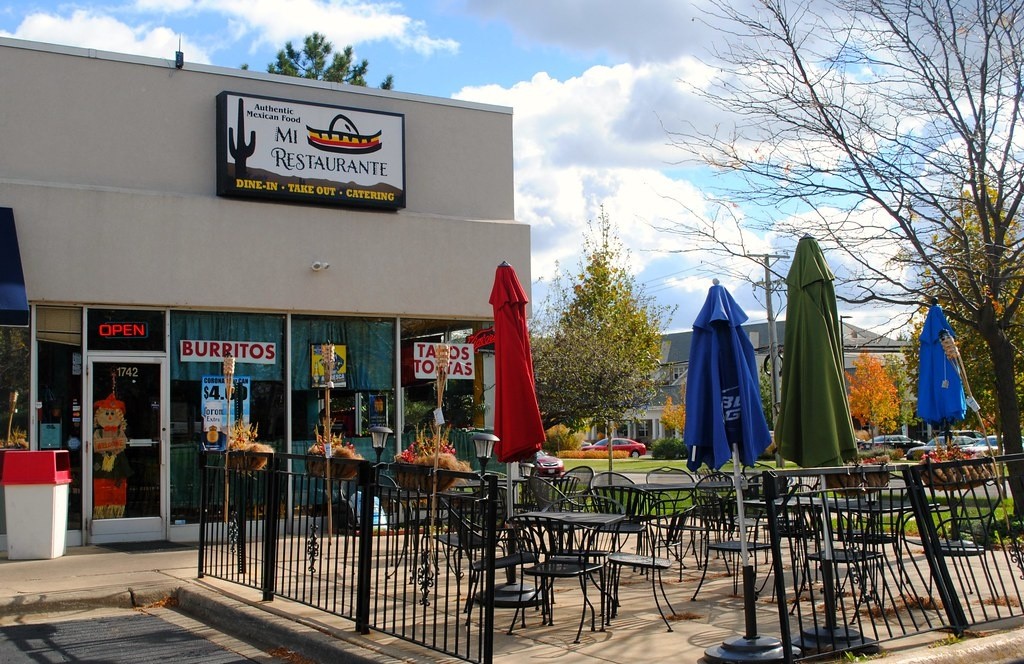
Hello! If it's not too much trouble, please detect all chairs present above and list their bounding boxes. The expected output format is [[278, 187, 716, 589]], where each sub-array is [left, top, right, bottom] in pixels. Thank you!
[[376, 461, 1004, 646]]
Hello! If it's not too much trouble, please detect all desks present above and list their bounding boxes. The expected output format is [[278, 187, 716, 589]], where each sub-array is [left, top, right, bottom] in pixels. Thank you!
[[620, 482, 763, 569], [730, 494, 912, 625], [507, 511, 627, 644], [497, 473, 569, 539], [421, 479, 506, 580]]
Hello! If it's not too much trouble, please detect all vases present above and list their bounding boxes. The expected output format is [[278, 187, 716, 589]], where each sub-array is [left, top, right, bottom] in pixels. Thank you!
[[224, 450, 268, 471], [394, 463, 455, 493], [306, 455, 358, 479], [824, 465, 891, 494], [919, 460, 995, 492]]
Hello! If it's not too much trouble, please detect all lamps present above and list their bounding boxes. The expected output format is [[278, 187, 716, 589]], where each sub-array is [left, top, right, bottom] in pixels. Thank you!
[[311, 262, 330, 272], [367, 426, 394, 454], [470, 431, 500, 466]]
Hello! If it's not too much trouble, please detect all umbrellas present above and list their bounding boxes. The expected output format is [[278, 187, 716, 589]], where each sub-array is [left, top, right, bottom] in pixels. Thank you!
[[474, 258, 547, 609], [916, 296, 986, 557], [683, 231, 880, 664]]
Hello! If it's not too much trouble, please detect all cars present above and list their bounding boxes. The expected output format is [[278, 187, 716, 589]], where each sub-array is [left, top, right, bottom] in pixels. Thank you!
[[952, 434, 1024, 455], [855, 434, 926, 455], [535, 449, 565, 478], [581, 437, 646, 459], [905, 436, 976, 461]]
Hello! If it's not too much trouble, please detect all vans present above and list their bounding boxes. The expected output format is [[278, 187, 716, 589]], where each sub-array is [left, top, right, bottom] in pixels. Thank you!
[[938, 429, 986, 443]]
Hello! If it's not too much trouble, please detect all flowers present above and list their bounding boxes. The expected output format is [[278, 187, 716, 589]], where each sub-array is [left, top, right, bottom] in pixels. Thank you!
[[0, 427, 30, 449], [394, 420, 472, 474], [844, 454, 889, 466], [914, 426, 990, 460], [309, 416, 362, 460], [219, 419, 273, 452]]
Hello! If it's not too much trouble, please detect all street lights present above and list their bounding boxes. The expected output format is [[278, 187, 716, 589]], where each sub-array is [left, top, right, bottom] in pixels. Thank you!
[[839, 314, 854, 360]]
